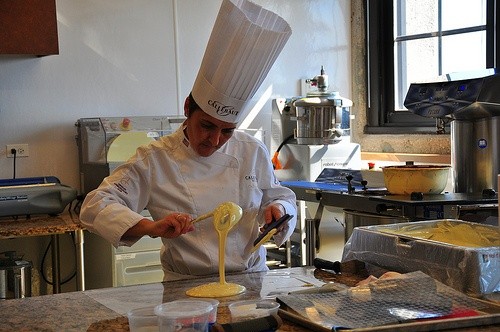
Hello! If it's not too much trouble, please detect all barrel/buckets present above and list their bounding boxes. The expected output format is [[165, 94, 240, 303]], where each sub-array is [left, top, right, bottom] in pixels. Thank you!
[[449, 115, 500, 194], [0, 250, 33, 300]]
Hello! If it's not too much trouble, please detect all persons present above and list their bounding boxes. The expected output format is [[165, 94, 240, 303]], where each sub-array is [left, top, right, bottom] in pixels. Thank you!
[[80, 81, 297, 282]]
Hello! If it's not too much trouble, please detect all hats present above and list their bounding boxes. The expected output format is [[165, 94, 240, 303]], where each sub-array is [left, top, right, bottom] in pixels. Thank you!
[[190, 1, 292, 125]]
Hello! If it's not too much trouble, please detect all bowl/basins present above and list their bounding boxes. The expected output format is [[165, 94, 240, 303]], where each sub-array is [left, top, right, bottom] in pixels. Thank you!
[[126, 297, 220, 332], [229, 299, 280, 322], [379, 164, 452, 195]]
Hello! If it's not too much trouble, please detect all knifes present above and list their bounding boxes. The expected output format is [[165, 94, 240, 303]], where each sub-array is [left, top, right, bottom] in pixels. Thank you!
[[314, 258, 366, 275]]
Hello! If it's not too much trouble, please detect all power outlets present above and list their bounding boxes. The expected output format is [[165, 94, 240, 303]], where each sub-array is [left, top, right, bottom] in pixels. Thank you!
[[6, 144, 29, 157]]
